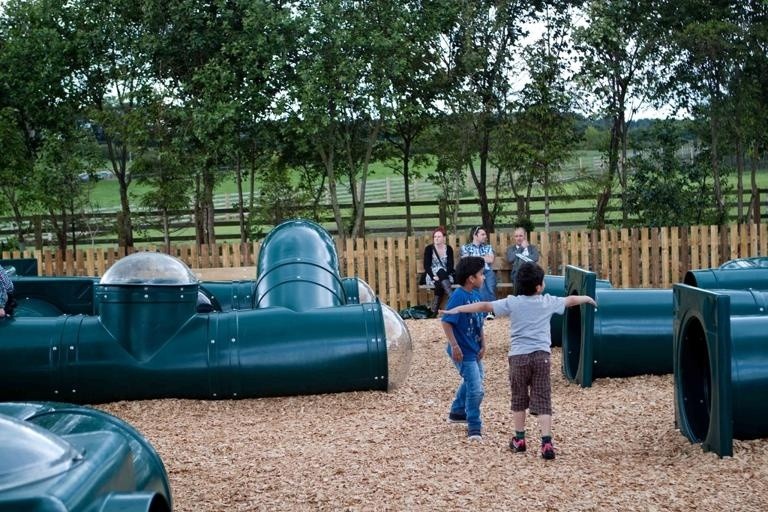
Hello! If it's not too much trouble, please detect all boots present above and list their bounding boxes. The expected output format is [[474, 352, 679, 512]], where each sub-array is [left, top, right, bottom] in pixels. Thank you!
[[440, 279, 453, 301], [427, 294, 442, 318]]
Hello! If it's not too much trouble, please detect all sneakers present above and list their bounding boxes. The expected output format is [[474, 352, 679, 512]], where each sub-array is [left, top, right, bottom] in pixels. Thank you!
[[541, 443, 555, 460], [508, 436, 526, 453], [468, 430, 482, 443], [486, 311, 495, 320], [446, 413, 467, 424]]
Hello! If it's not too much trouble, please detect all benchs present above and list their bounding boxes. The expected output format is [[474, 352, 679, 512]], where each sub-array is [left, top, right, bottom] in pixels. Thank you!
[[413, 255, 545, 310]]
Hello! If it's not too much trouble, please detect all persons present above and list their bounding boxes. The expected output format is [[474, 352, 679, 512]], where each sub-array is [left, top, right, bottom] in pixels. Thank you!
[[459, 225, 497, 320], [0, 265, 17, 318], [506, 227, 540, 297], [420, 225, 453, 318], [441, 256, 486, 441], [438, 261, 598, 459]]
